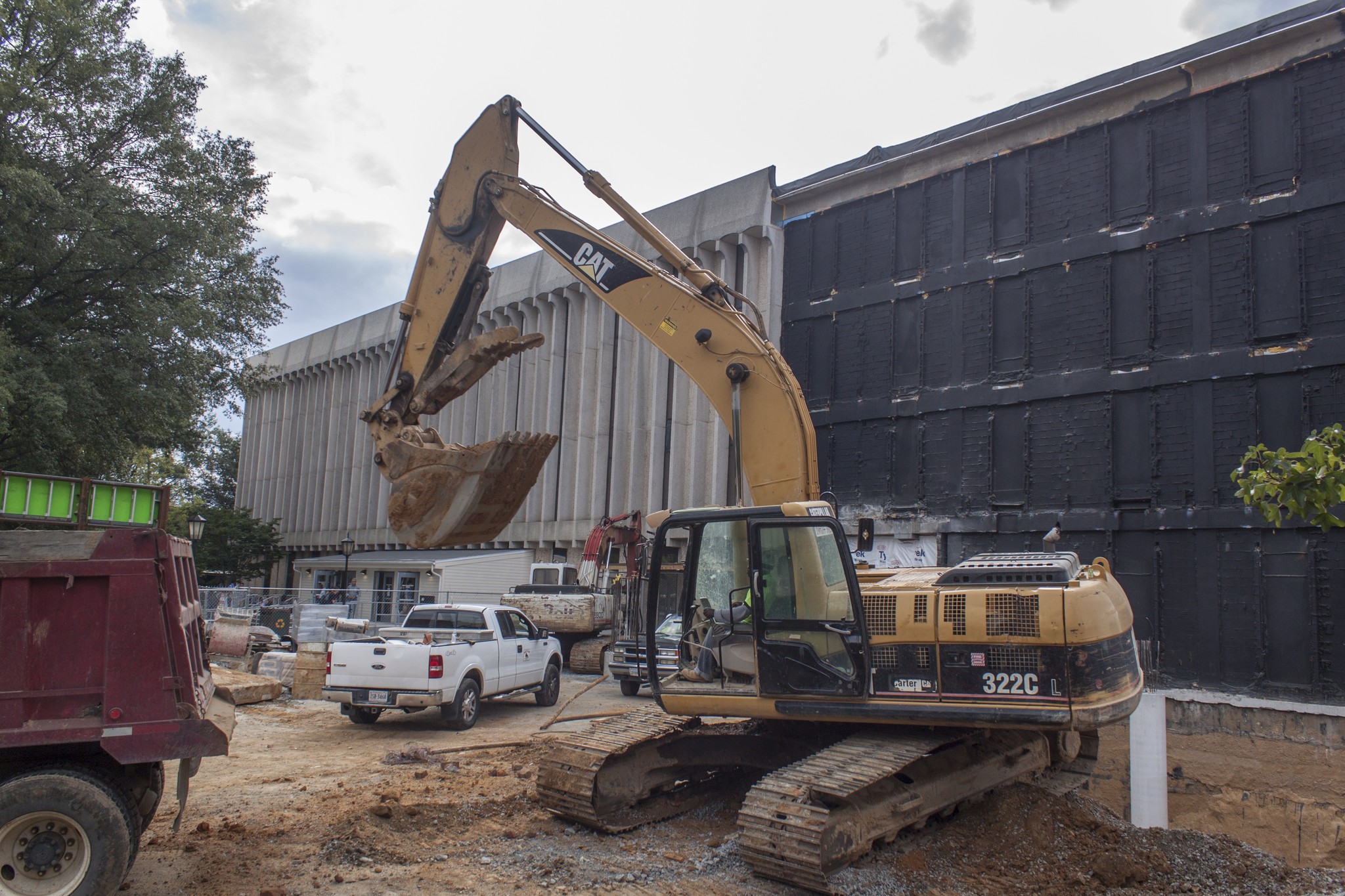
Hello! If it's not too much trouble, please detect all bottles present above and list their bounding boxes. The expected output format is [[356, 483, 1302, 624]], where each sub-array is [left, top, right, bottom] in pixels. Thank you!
[[451, 629, 457, 643]]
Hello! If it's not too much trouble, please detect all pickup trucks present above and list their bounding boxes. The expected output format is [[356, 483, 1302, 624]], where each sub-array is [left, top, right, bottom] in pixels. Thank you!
[[321, 603, 565, 730]]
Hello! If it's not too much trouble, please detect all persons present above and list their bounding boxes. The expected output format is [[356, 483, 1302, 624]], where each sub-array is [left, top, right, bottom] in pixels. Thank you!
[[226, 580, 270, 613], [682, 547, 778, 684], [318, 578, 361, 618]]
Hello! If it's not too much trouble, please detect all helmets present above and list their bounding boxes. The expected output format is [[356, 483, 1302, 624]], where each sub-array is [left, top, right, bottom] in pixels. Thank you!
[[746, 547, 774, 566]]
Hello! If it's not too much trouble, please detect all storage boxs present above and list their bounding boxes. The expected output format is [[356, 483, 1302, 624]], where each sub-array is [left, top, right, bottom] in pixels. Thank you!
[[252, 601, 372, 702]]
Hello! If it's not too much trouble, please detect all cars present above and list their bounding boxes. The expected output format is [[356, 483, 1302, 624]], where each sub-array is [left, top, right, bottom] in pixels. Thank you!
[[609, 610, 683, 697]]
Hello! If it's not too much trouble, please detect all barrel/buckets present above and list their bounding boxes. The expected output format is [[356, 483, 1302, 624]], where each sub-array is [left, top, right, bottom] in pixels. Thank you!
[[291, 651, 328, 700]]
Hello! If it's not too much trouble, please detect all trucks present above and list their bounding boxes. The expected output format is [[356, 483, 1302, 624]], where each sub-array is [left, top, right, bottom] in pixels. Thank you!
[[0, 469, 236, 896]]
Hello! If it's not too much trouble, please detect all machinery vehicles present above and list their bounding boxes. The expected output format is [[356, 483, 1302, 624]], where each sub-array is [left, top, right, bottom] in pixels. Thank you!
[[356, 93, 1147, 896], [497, 508, 650, 675]]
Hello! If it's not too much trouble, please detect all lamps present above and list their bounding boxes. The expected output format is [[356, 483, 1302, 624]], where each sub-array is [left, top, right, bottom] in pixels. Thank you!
[[305, 568, 312, 574], [425, 570, 433, 576], [360, 569, 367, 575]]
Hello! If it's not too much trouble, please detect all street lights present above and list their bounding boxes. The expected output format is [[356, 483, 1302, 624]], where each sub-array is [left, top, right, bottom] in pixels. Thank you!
[[188, 509, 207, 563], [340, 532, 356, 604]]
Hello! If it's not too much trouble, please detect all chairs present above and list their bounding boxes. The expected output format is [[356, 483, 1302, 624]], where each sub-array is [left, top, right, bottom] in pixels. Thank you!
[[433, 619, 454, 629], [734, 553, 793, 637]]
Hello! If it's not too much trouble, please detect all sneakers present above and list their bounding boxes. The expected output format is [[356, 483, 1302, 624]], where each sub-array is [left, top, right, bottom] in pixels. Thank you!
[[682, 668, 708, 683]]
